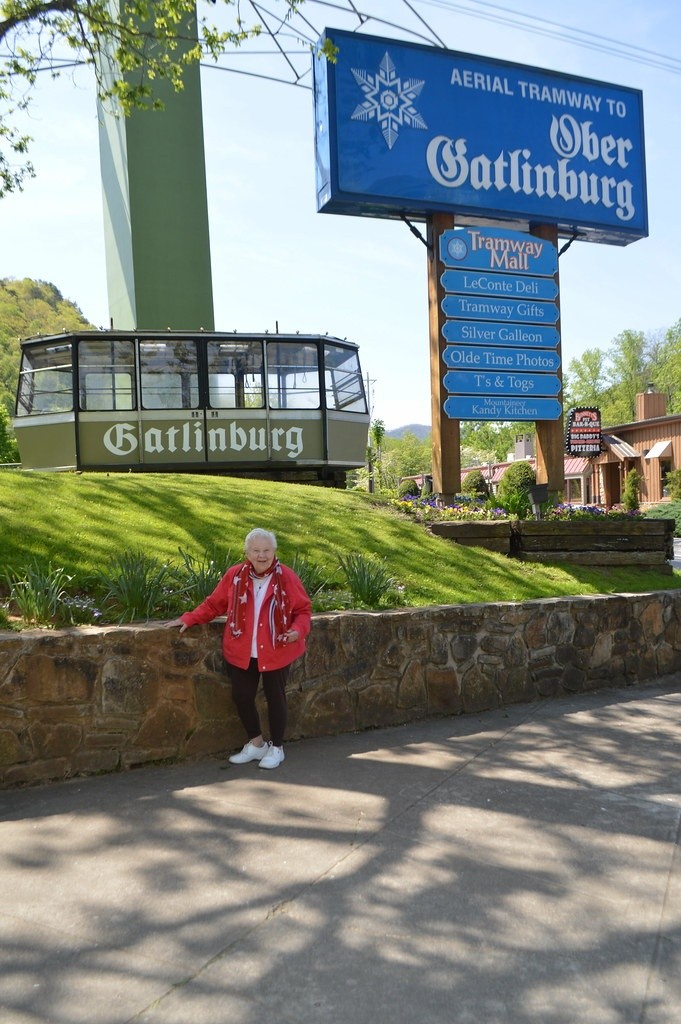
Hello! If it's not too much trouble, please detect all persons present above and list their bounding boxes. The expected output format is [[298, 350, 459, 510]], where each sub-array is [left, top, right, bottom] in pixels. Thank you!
[[162, 528, 313, 770]]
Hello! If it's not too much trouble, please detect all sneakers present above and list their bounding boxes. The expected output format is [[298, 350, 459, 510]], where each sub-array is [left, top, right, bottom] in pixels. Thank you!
[[259, 740, 285, 769], [228, 741, 268, 764]]
